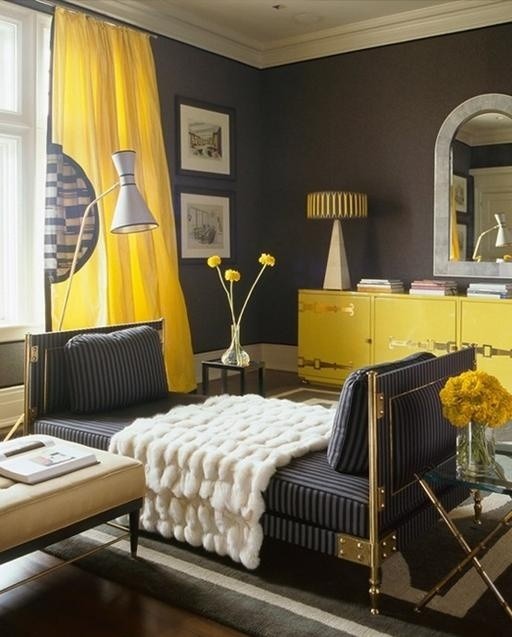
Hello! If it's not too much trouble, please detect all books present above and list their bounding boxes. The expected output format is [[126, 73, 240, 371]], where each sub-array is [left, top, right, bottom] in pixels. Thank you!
[[467, 283, 512, 300], [357, 279, 404, 293], [0, 435, 101, 487], [410, 280, 458, 295]]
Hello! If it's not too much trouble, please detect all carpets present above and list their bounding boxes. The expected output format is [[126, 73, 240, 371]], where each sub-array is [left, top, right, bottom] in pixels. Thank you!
[[42, 383, 512, 637]]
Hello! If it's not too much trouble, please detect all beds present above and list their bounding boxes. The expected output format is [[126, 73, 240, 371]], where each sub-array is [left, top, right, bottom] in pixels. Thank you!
[[24, 317, 482, 615]]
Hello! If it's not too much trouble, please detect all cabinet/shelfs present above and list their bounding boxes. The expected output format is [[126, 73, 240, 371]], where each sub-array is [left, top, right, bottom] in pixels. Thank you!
[[461, 301, 512, 396], [297, 294, 370, 381], [374, 297, 456, 368]]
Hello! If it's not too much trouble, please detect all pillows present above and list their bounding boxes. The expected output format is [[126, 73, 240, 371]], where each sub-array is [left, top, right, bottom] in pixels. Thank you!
[[64, 326, 169, 410], [329, 352, 437, 472]]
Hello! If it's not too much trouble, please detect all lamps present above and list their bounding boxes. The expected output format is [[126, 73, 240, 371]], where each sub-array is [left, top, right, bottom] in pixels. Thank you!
[[307, 191, 367, 291], [1, 149, 159, 442], [472, 213, 512, 261]]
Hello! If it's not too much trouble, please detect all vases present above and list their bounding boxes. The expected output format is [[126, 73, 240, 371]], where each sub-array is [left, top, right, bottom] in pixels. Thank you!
[[456, 419, 495, 476], [222, 325, 249, 367]]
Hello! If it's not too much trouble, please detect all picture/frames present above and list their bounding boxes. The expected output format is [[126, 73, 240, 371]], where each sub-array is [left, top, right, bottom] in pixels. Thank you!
[[175, 186, 237, 266], [174, 96, 237, 182]]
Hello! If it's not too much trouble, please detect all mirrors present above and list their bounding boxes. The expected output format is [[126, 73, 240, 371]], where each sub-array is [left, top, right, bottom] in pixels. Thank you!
[[432, 92, 512, 279]]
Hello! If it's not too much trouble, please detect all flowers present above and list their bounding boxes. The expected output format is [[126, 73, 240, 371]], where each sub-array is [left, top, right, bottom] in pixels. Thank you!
[[439, 369, 512, 428], [209, 253, 275, 324]]
